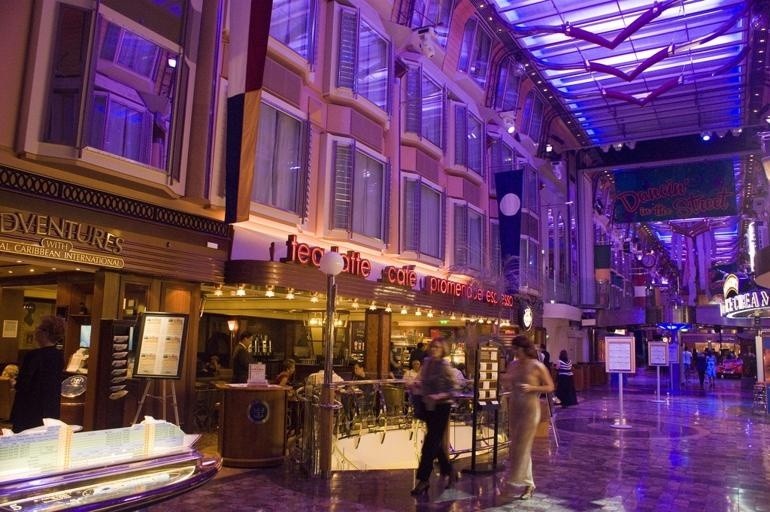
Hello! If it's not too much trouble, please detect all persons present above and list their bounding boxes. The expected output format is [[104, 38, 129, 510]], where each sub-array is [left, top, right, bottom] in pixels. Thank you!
[[499, 333, 557, 501], [10, 317, 67, 435], [410, 338, 462, 497], [554, 347, 579, 408], [683, 345, 719, 386], [537, 341, 548, 396], [196, 330, 474, 421]]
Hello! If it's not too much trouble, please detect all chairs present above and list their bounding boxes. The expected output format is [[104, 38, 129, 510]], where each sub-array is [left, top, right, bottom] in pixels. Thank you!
[[278, 372, 494, 453]]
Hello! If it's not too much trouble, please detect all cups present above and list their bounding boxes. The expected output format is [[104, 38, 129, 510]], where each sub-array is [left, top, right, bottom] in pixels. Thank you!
[[425, 397, 436, 411]]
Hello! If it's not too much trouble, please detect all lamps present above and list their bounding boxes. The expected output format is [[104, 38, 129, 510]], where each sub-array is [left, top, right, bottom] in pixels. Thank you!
[[335, 296, 434, 319], [418, 27, 437, 60], [211, 282, 320, 304], [551, 161, 562, 182], [499, 112, 516, 135], [438, 310, 512, 328]]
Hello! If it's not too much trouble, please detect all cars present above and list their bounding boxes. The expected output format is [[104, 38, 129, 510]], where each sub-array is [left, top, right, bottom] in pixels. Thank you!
[[716, 356, 745, 377]]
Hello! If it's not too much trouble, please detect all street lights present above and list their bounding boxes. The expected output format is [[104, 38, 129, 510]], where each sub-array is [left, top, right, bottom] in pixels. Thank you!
[[315, 249, 345, 479]]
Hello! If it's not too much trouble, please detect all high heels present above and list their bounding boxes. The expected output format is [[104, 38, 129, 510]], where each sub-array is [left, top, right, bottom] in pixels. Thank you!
[[410, 481, 430, 495], [444, 469, 459, 490], [520, 485, 535, 500]]
